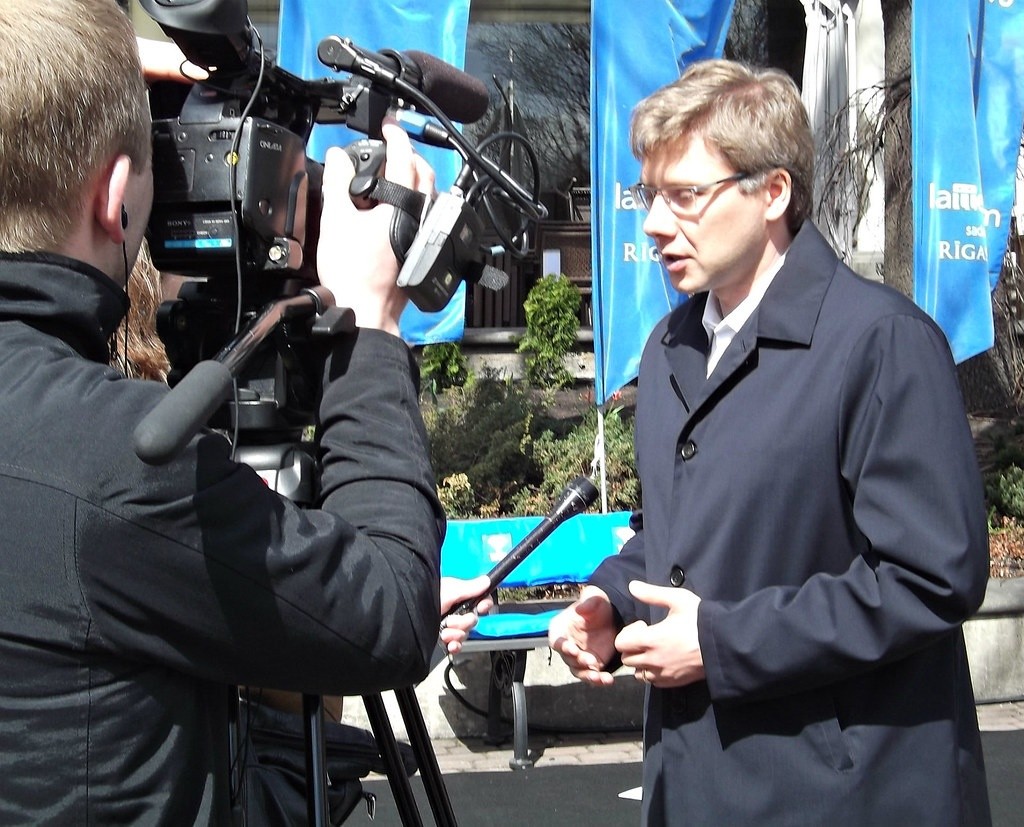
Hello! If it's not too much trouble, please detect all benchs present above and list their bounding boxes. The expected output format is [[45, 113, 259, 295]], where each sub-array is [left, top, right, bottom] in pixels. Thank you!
[[442, 509, 639, 773]]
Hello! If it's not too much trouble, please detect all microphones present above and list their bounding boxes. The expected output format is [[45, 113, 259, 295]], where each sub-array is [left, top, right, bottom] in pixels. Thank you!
[[435, 479, 600, 636], [315, 36, 489, 126]]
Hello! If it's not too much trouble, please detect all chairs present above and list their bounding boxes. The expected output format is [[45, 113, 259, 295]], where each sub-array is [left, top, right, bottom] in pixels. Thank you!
[[529, 178, 591, 328]]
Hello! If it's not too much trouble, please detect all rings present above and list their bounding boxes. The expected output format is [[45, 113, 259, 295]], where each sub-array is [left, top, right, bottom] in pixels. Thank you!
[[642, 668, 653, 685]]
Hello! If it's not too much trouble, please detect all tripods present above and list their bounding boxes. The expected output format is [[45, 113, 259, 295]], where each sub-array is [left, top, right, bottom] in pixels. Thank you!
[[132, 278, 460, 827]]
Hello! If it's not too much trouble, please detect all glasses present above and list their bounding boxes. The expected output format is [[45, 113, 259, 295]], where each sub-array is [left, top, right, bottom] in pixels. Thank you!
[[627, 168, 757, 214]]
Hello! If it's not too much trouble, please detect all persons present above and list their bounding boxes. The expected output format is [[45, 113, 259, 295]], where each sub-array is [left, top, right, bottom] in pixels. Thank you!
[[0, 0, 446, 827], [548, 61, 989, 827]]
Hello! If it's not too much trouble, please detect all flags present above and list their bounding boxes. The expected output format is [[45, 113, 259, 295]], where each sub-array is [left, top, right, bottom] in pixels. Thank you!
[[911, 0, 1024, 364], [278, 0, 472, 345], [592, 0, 739, 398]]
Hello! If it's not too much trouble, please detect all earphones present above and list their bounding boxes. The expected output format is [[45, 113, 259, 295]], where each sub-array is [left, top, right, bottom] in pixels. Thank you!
[[120, 204, 129, 231]]
